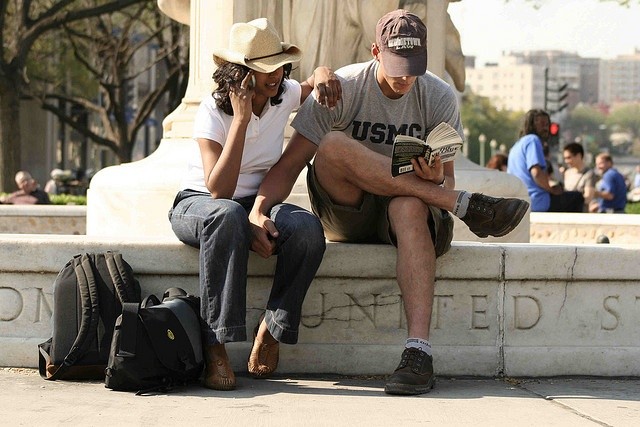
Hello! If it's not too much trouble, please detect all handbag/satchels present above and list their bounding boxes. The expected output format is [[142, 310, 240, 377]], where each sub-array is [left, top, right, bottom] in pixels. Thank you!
[[103, 287, 205, 396]]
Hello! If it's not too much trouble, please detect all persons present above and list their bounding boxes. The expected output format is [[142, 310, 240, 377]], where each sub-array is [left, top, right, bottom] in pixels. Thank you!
[[0, 169, 51, 204], [590, 153, 627, 213], [168, 18, 342, 390], [564, 143, 595, 213], [248, 9, 530, 395], [506, 109, 584, 213], [44, 168, 72, 194], [486, 154, 508, 172], [543, 145, 553, 174], [626, 165, 640, 203]]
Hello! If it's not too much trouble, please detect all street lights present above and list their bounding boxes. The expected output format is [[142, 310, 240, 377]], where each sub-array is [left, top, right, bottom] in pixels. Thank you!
[[478, 133, 487, 166], [489, 139, 497, 157], [463, 128, 469, 157]]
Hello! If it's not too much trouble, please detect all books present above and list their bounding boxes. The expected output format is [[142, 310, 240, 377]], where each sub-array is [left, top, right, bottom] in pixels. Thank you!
[[390, 121, 464, 178]]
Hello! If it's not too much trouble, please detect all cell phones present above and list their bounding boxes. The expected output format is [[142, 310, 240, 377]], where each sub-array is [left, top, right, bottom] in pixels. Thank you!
[[248, 75, 256, 88]]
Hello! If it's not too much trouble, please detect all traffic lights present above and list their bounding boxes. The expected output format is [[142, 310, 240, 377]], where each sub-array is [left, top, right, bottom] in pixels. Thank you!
[[549, 122, 560, 146]]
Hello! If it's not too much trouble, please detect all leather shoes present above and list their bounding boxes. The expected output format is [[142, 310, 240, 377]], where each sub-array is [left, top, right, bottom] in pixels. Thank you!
[[463, 193, 529, 237], [202, 358, 237, 392], [247, 313, 280, 378], [385, 347, 435, 394]]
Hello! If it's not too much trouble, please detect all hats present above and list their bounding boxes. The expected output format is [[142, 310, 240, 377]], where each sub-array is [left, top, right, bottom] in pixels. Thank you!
[[376, 10, 427, 77], [213, 18, 303, 73]]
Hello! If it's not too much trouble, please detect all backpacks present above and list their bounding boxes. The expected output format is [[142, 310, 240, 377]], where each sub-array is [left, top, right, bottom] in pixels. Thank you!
[[38, 252, 142, 380]]
